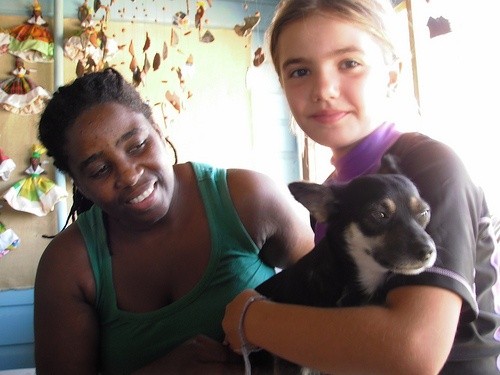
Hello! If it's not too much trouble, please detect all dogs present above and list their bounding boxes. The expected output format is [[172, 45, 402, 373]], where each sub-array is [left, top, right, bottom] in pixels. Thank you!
[[236, 153, 437, 375]]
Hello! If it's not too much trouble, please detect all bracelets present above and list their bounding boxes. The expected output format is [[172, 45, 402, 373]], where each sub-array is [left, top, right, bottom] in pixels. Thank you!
[[238, 295, 265, 374]]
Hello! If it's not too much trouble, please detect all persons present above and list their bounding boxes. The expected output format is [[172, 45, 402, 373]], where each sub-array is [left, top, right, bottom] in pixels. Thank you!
[[33, 68, 316, 374], [222, 1, 500, 375]]
